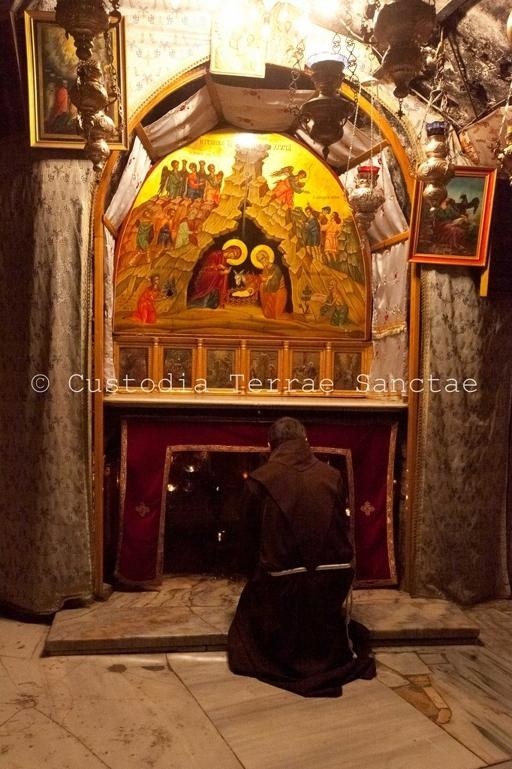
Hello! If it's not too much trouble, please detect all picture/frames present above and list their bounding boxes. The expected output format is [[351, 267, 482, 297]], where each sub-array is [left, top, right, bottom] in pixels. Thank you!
[[24, 9, 129, 151], [406, 158, 497, 268]]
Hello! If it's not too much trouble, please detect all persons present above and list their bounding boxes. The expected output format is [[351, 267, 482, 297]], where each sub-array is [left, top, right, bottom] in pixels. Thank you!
[[125, 153, 363, 328], [226, 417, 376, 697]]
